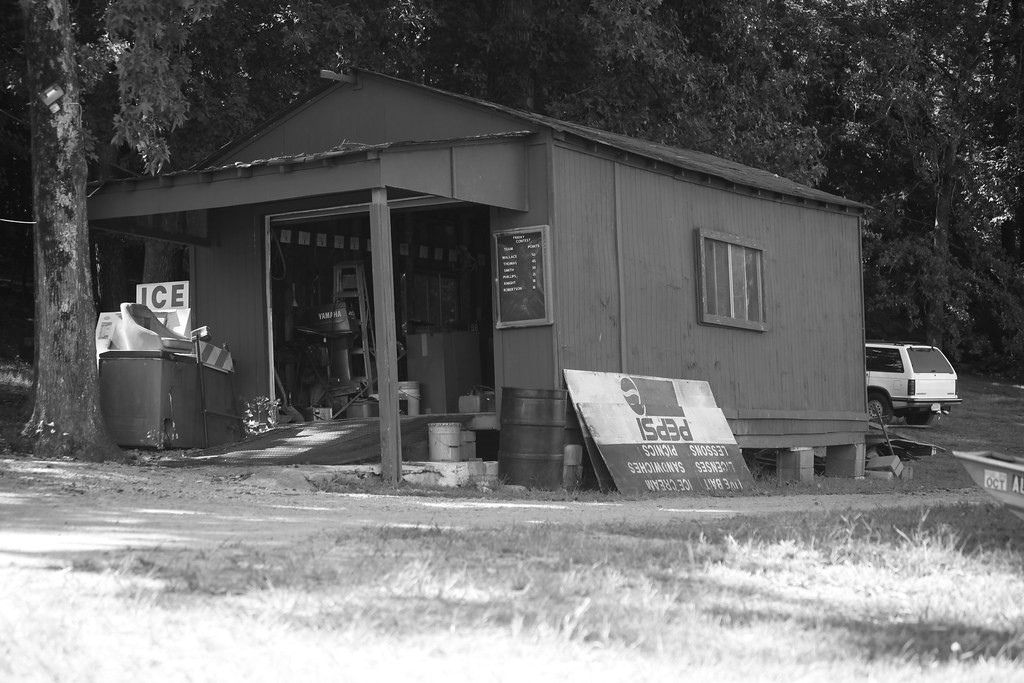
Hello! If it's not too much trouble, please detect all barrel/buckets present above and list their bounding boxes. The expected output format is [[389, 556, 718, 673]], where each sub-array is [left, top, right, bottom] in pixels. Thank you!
[[498, 386, 569, 491], [398, 381, 421, 415], [427, 422, 462, 462]]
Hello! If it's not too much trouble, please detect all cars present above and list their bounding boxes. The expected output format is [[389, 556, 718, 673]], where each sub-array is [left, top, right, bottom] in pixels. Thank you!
[[1, 279, 35, 363]]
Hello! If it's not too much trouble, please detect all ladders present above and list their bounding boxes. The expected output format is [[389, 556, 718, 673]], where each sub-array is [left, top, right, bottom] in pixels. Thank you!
[[332, 260, 376, 397]]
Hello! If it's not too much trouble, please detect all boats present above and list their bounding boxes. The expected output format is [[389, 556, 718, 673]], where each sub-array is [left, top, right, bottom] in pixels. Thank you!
[[952, 448, 1024, 522]]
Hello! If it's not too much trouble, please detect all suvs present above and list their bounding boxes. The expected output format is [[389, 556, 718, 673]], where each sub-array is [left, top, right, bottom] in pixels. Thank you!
[[865, 339, 962, 426]]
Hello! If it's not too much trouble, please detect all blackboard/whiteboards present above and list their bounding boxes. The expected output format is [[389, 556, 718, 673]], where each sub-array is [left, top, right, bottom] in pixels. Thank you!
[[492, 225, 552, 329]]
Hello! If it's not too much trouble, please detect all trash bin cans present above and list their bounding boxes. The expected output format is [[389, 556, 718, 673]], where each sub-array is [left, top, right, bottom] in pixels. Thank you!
[[98, 351, 228, 450]]
